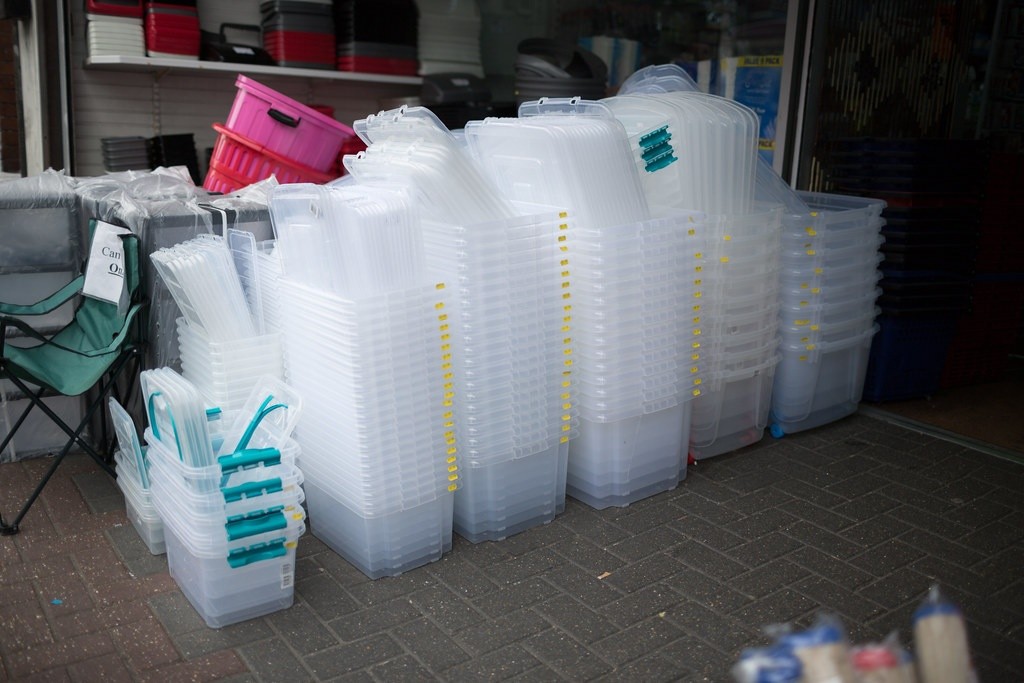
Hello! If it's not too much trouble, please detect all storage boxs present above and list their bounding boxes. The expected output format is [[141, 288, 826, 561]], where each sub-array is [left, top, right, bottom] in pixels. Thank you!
[[1, 1, 981, 630]]
